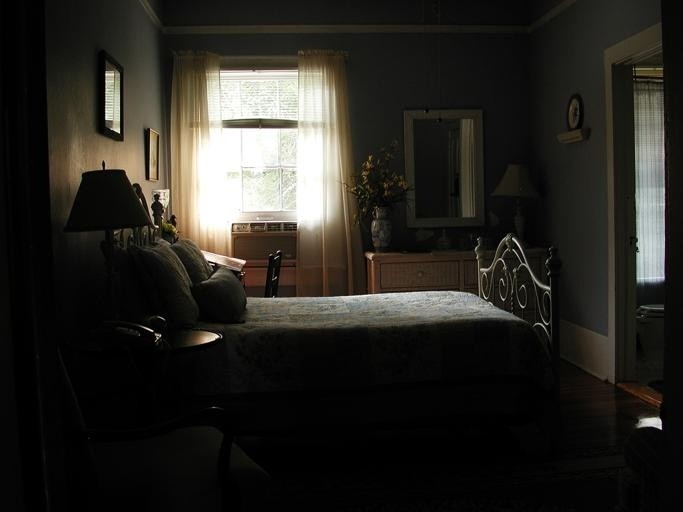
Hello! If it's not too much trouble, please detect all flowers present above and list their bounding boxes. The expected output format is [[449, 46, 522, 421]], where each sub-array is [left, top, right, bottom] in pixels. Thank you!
[[340, 139, 417, 226]]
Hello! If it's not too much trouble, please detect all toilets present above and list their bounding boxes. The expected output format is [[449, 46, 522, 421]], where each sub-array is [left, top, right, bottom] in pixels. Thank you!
[[636, 303, 665, 387]]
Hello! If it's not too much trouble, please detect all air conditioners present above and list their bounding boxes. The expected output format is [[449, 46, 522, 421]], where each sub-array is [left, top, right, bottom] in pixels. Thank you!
[[227, 219, 299, 268]]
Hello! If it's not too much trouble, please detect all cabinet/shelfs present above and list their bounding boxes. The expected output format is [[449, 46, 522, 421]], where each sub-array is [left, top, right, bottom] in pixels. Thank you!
[[363, 250, 557, 353]]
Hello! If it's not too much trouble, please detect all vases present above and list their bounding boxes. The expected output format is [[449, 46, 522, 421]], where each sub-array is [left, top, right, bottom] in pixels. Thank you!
[[369, 208, 391, 249]]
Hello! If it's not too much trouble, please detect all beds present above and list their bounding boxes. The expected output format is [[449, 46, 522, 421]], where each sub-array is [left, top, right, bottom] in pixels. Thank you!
[[56, 224, 576, 506]]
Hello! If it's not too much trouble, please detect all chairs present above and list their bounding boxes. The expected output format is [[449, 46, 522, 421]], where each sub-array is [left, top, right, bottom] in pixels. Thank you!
[[263, 250, 280, 297]]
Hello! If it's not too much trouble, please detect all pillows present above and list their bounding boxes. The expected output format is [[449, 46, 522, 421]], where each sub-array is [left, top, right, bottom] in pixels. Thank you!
[[95, 237, 247, 324]]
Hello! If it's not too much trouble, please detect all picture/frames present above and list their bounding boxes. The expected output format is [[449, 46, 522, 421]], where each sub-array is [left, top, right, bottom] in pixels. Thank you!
[[145, 128, 161, 181], [96, 49, 123, 141]]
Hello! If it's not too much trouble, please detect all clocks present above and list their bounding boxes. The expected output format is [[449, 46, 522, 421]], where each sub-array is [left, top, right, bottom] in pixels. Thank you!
[[566, 93, 584, 131]]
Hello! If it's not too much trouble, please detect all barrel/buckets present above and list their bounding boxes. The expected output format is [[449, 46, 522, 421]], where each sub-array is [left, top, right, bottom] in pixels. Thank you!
[[126, 327, 230, 418]]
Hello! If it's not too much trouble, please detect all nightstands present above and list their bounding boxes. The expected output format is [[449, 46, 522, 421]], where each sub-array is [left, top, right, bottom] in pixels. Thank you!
[[70, 322, 235, 512]]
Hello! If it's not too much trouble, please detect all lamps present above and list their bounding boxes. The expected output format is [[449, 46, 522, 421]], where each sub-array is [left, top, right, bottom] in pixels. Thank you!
[[64, 158, 154, 291], [150, 189, 169, 219]]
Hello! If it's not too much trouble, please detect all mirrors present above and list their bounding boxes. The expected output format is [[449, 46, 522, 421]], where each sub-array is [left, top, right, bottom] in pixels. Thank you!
[[402, 110, 491, 228]]
